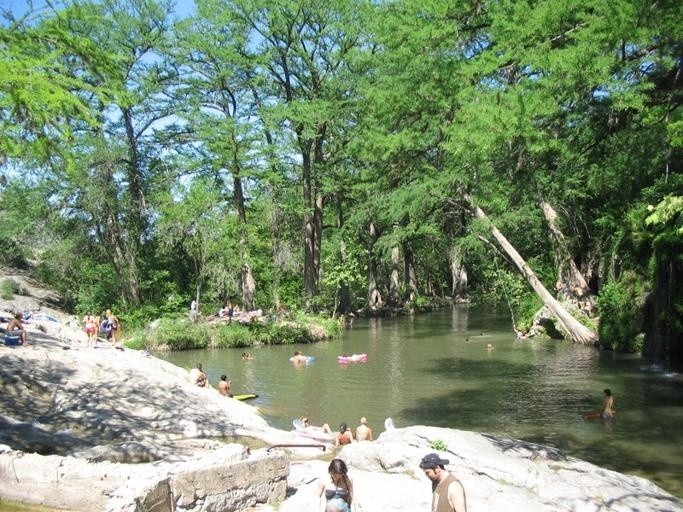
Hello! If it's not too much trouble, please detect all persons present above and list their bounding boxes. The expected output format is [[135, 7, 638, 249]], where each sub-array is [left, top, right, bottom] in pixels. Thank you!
[[8, 307, 34, 320], [244, 352, 247, 358], [83, 308, 120, 348], [6, 311, 29, 346], [420, 454, 466, 512], [189, 298, 197, 312], [335, 422, 354, 446], [294, 350, 310, 361], [315, 458, 357, 512], [600, 388, 615, 418], [220, 375, 233, 397], [213, 301, 289, 327], [355, 417, 372, 441], [189, 364, 208, 387], [485, 343, 494, 350], [241, 355, 245, 359], [552, 280, 565, 302], [572, 284, 586, 309], [343, 352, 369, 359], [299, 416, 333, 434], [246, 355, 252, 359]]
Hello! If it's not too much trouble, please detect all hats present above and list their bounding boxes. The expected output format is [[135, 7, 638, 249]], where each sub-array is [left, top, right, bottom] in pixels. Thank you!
[[420, 452, 450, 467]]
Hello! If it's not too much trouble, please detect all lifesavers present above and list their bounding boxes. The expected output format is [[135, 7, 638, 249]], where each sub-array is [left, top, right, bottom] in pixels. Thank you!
[[100, 320, 112, 333]]
